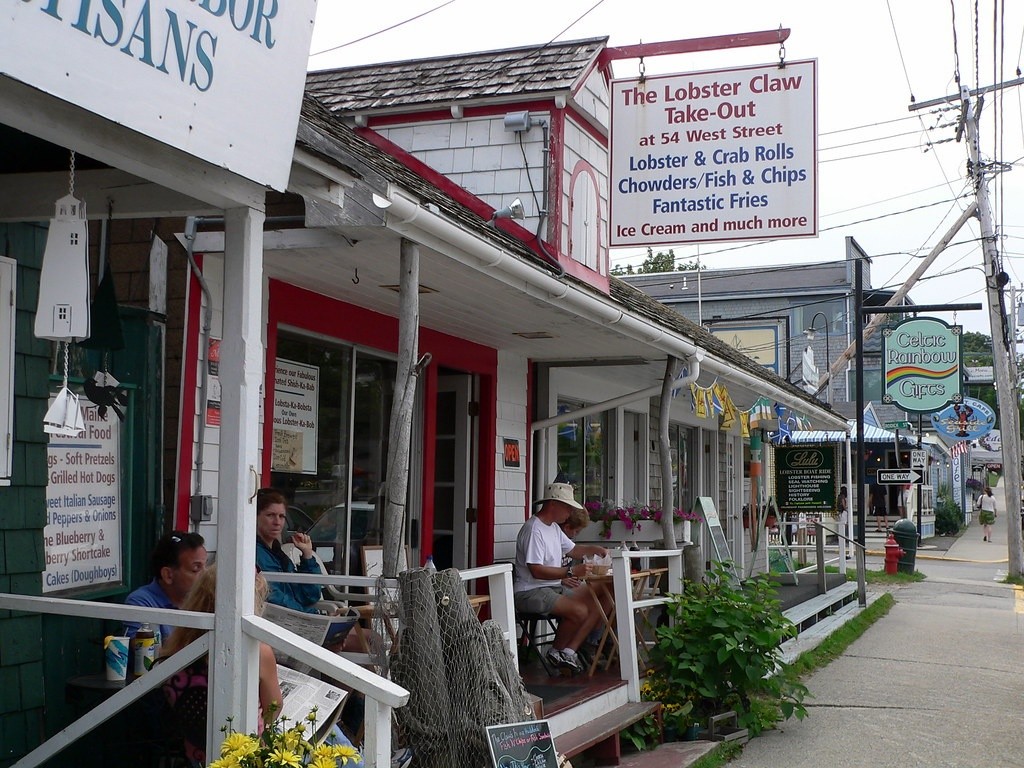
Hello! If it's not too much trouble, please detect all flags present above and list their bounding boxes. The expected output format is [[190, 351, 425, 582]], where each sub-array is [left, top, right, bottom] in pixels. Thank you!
[[672, 367, 813, 446]]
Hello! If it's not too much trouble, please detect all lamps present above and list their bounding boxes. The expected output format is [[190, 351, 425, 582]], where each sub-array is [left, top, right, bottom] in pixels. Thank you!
[[486, 198, 527, 229], [504, 110, 532, 131], [808, 312, 832, 409]]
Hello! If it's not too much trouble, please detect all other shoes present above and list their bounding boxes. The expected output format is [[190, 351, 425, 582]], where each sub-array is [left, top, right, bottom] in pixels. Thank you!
[[600, 637, 618, 666], [984, 536, 987, 541], [579, 640, 603, 670]]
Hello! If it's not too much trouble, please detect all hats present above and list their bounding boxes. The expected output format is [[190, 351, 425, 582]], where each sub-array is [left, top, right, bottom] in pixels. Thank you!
[[533, 482, 583, 509]]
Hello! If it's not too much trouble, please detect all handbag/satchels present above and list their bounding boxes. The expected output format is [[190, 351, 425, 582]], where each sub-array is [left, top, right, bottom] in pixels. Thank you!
[[976, 495, 983, 508]]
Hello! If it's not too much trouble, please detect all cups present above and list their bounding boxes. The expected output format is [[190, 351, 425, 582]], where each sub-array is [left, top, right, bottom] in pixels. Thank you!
[[105, 636, 130, 681]]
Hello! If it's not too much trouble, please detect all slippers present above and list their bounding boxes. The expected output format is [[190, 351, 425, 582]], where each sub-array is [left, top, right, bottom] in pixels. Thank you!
[[886, 527, 890, 529], [875, 529, 882, 532]]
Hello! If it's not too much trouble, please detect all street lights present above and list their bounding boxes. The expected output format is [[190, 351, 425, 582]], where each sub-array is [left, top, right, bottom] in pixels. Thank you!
[[806, 312, 830, 405]]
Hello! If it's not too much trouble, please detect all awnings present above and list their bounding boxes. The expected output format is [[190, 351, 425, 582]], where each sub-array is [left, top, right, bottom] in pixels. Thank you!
[[770, 422, 930, 445]]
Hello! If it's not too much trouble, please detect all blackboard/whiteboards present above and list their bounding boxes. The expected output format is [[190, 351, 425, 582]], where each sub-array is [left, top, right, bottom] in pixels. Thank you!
[[697, 495, 745, 597], [361, 543, 414, 654], [41, 391, 125, 592], [483, 719, 560, 768]]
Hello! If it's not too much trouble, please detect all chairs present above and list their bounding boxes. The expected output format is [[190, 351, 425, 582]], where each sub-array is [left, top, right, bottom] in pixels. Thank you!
[[492, 560, 591, 677], [148, 654, 210, 768], [280, 543, 392, 619]]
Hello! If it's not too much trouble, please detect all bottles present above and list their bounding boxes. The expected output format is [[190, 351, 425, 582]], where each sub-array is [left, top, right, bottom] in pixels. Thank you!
[[133, 623, 154, 678], [154, 623, 162, 667], [423, 555, 437, 573], [629, 540, 641, 572], [620, 540, 628, 551]]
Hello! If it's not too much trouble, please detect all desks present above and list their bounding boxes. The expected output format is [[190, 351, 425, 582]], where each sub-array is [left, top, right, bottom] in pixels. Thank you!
[[335, 595, 490, 748], [63, 674, 133, 700], [575, 568, 670, 677]]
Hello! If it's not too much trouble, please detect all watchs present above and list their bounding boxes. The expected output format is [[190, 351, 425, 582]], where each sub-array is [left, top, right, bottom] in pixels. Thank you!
[[567, 565, 573, 577]]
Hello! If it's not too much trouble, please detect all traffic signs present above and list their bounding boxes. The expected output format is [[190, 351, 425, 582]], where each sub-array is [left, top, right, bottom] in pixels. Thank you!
[[877, 469, 924, 484], [910, 450, 927, 471]]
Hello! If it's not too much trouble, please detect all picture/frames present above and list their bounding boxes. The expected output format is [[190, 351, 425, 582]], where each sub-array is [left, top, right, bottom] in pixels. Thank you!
[[361, 545, 412, 642]]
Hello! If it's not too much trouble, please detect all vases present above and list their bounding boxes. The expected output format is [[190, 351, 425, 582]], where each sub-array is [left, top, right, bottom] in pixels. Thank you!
[[765, 515, 776, 527], [573, 519, 691, 542], [743, 516, 758, 528], [967, 487, 979, 493]]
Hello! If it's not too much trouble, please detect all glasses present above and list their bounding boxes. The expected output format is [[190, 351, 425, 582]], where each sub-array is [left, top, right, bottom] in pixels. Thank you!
[[257, 488, 281, 496], [164, 533, 188, 563]]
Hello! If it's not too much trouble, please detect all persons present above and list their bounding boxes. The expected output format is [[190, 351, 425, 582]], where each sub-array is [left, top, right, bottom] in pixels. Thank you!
[[122, 531, 283, 768], [897, 485, 910, 519], [977, 487, 998, 542], [513, 483, 612, 674], [837, 486, 851, 560], [256, 489, 389, 749], [868, 481, 890, 532]]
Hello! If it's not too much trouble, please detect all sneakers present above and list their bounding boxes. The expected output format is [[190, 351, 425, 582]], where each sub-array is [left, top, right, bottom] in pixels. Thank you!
[[391, 748, 413, 768], [546, 647, 584, 672]]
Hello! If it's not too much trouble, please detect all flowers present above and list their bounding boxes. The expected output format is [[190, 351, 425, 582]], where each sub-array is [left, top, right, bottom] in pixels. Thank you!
[[743, 503, 760, 517], [199, 700, 363, 768], [966, 478, 983, 491], [585, 499, 701, 541], [762, 504, 777, 517]]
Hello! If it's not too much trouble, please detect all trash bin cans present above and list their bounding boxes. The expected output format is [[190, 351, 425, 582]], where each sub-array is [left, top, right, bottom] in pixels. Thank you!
[[888, 519, 920, 572]]
